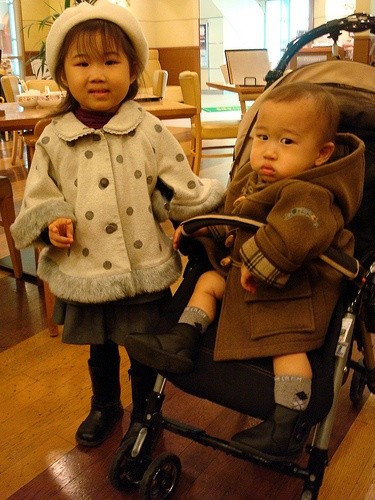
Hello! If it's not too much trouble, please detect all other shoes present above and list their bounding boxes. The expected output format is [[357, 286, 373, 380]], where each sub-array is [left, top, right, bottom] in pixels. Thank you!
[[231, 419, 303, 459], [124, 322, 197, 373]]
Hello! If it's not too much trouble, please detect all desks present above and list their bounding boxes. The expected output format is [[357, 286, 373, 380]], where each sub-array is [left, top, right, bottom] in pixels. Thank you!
[[206, 81, 265, 93], [0, 98, 196, 128]]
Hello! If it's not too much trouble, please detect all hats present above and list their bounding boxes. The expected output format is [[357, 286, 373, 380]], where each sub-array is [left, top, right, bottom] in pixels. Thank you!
[[47, 0, 148, 81]]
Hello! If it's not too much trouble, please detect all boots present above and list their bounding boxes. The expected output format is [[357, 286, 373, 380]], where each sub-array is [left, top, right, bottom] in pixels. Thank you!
[[76, 355, 123, 445], [119, 369, 165, 454]]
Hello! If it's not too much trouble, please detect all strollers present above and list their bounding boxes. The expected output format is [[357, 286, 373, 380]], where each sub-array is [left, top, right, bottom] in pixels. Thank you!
[[105, 11, 373, 500]]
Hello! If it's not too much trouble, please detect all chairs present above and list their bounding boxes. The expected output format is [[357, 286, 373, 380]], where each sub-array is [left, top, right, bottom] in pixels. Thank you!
[[0, 49, 244, 336]]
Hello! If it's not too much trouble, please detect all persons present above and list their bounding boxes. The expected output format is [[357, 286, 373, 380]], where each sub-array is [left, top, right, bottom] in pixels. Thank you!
[[125, 82, 364, 461], [9, 0, 225, 448]]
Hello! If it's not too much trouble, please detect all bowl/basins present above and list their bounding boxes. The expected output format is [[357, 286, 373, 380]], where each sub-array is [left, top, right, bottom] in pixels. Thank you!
[[16, 90, 67, 109]]
